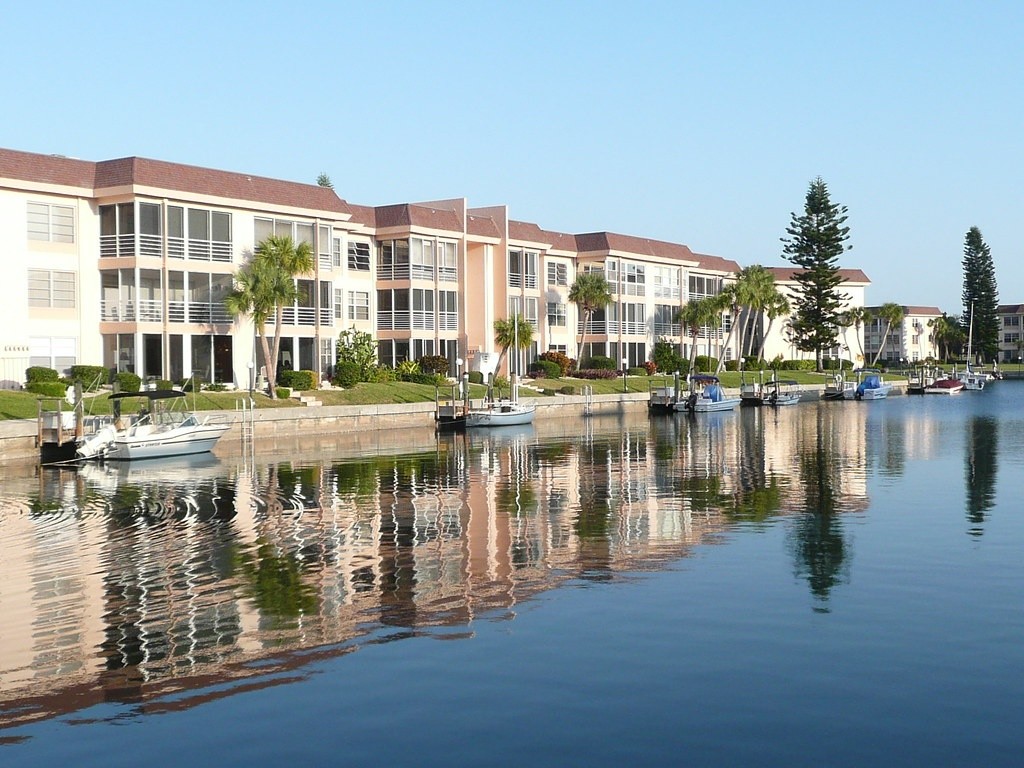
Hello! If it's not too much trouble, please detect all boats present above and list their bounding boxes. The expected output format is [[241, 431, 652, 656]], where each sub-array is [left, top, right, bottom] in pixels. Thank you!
[[924, 378, 965, 395], [759, 380, 803, 406], [842, 366, 893, 400], [672, 374, 743, 413], [75, 370, 233, 462]]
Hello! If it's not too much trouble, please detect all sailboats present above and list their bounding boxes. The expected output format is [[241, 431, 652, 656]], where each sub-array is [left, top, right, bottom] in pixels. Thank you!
[[456, 297, 536, 428], [959, 300, 985, 391]]
